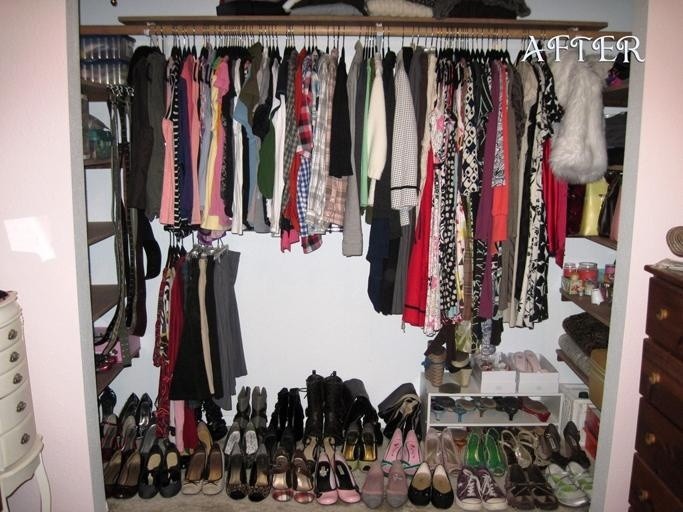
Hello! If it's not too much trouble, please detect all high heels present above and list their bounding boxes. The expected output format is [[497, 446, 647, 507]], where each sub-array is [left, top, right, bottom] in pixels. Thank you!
[[158, 443, 182, 498], [450, 399, 476, 423], [136, 392, 152, 436], [119, 415, 138, 462], [138, 443, 163, 499], [114, 451, 143, 499], [563, 421, 591, 469], [226, 441, 247, 500], [222, 421, 242, 470], [481, 428, 505, 477], [449, 361, 473, 387], [518, 430, 543, 466], [331, 452, 360, 503], [493, 396, 518, 421], [381, 428, 404, 477], [385, 465, 407, 508], [359, 423, 378, 472], [324, 436, 335, 471], [103, 451, 124, 500], [430, 397, 447, 421], [341, 422, 360, 471], [423, 340, 447, 387], [100, 385, 116, 436], [101, 414, 119, 464], [441, 488, 444, 490], [202, 443, 224, 495], [497, 430, 522, 468], [196, 418, 213, 454], [362, 464, 384, 508], [270, 448, 292, 502], [544, 424, 568, 469], [303, 435, 317, 462], [314, 452, 338, 505], [290, 450, 314, 504], [424, 428, 443, 475], [430, 464, 454, 509], [464, 432, 481, 467], [118, 392, 139, 429], [470, 396, 496, 417], [180, 444, 206, 495], [408, 461, 432, 507], [402, 430, 423, 476], [247, 444, 271, 502], [138, 423, 158, 469], [441, 428, 462, 477], [244, 422, 259, 469]]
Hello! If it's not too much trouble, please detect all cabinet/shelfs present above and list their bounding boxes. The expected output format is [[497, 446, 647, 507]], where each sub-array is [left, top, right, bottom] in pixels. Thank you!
[[81, 85, 141, 398], [0, 290, 50, 511], [556, 75, 630, 408], [419, 371, 564, 462], [627, 265, 683, 512]]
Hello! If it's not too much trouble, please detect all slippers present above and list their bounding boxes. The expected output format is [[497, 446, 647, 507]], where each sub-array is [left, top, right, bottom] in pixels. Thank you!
[[525, 350, 549, 374], [514, 351, 529, 372]]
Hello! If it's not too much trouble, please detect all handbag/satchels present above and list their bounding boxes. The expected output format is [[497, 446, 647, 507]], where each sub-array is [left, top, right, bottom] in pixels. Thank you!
[[609, 186, 622, 241], [598, 172, 622, 237], [577, 175, 609, 237]]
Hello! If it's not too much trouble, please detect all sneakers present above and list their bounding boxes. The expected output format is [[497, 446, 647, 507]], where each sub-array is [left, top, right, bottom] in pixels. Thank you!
[[565, 461, 593, 503], [455, 465, 482, 510], [545, 464, 588, 507], [475, 465, 508, 511]]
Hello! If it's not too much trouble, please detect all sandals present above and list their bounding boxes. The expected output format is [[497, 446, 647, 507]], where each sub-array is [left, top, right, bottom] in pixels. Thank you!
[[518, 396, 551, 422], [523, 466, 558, 510], [504, 464, 534, 510]]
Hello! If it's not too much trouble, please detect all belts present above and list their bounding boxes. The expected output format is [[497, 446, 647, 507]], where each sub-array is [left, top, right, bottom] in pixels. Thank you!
[[94, 85, 138, 368]]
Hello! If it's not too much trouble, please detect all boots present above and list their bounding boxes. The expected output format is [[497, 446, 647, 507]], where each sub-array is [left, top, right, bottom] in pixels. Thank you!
[[322, 370, 342, 445], [282, 388, 303, 442], [233, 386, 251, 430], [250, 386, 267, 433], [302, 369, 323, 445], [268, 387, 289, 437], [341, 378, 383, 445], [378, 383, 422, 443]]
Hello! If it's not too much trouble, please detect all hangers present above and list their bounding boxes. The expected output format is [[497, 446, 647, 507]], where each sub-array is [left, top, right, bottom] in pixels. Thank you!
[[78, 24, 632, 85]]
